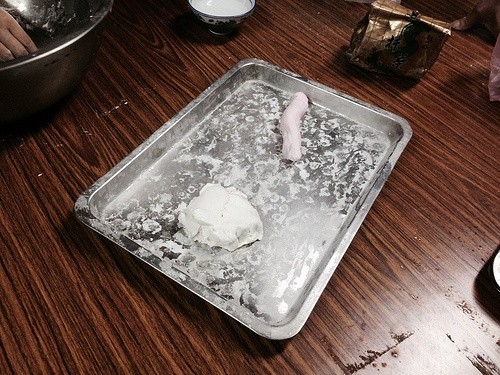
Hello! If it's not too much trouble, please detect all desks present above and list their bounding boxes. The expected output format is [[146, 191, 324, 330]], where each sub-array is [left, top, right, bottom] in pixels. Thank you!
[[0, 1, 500, 375]]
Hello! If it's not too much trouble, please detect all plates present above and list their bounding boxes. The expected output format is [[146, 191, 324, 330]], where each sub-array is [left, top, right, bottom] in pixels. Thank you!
[[74, 57, 413, 341], [492, 246, 500, 291]]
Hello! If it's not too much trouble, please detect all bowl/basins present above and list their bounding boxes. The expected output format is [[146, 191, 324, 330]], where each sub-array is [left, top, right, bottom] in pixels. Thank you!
[[187, 0, 256, 34], [0, 1, 114, 112]]
